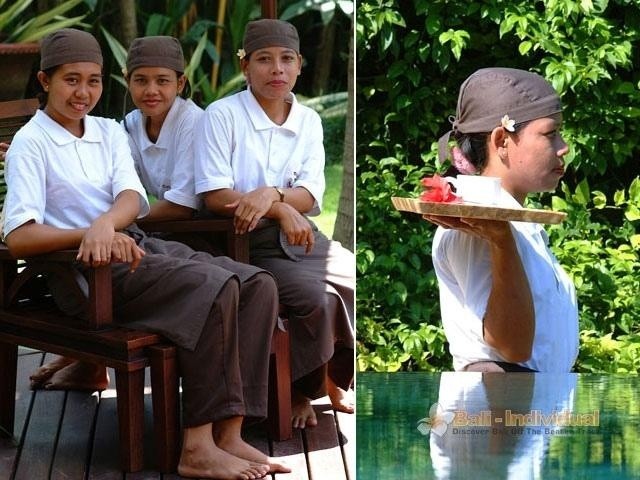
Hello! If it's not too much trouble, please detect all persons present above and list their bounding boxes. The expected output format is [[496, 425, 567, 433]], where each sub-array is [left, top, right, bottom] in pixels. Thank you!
[[431, 65, 580, 373], [194, 19, 354, 428], [28, 34, 206, 392], [1, 27, 291, 480], [429, 372, 578, 480]]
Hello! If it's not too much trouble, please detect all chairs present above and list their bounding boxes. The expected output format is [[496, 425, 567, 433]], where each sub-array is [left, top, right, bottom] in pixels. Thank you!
[[0, 99, 292, 474]]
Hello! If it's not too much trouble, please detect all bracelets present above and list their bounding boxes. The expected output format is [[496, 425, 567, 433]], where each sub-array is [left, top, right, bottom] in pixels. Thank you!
[[274, 185, 286, 201]]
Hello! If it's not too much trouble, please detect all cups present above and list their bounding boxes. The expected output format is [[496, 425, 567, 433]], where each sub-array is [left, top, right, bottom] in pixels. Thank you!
[[445, 175, 502, 204]]
